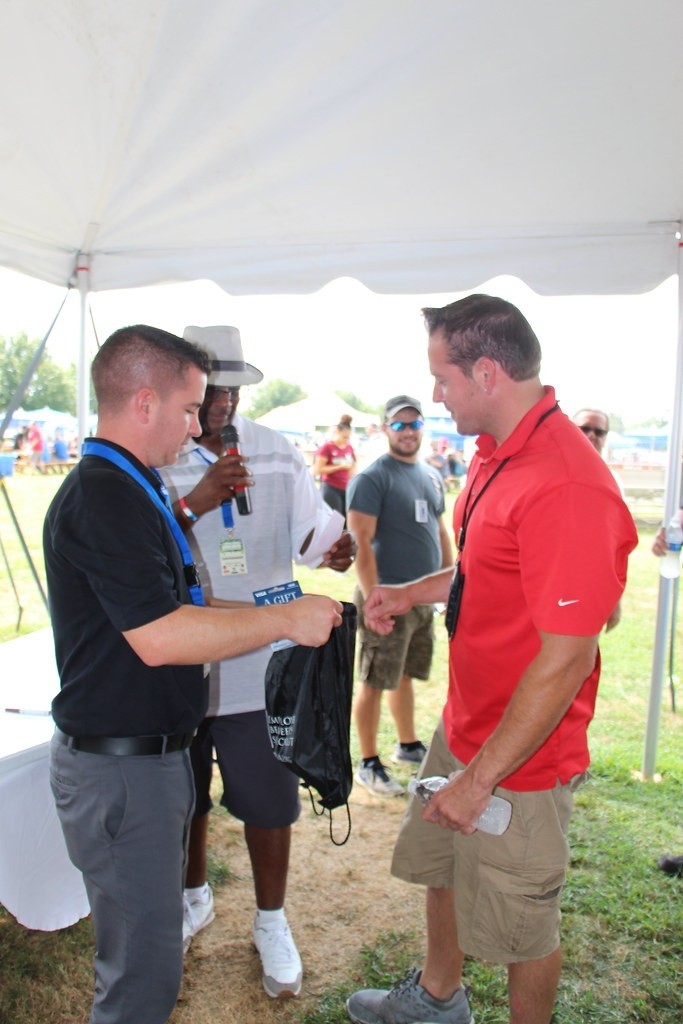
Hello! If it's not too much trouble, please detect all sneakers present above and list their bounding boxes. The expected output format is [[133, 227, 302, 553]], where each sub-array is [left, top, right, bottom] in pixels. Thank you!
[[346, 967, 475, 1024], [183, 887, 215, 956], [353, 762, 406, 797], [251, 912, 303, 1000], [390, 740, 429, 767]]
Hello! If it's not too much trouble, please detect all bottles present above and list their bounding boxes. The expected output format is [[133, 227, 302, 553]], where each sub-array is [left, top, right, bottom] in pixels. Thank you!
[[659, 519, 683, 578], [408, 776, 512, 834]]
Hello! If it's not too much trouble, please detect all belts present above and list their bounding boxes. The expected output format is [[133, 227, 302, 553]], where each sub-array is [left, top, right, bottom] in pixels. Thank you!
[[56, 725, 197, 757]]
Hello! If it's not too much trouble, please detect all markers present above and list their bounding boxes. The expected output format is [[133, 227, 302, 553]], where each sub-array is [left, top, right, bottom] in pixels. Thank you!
[[5, 708, 51, 716]]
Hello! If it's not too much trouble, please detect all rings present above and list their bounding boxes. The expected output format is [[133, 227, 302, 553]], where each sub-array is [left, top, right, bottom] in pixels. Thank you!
[[349, 555, 355, 561], [229, 485, 235, 496]]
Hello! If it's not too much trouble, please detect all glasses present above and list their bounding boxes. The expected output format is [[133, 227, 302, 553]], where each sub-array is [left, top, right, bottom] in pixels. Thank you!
[[385, 421, 424, 431], [205, 388, 241, 401], [578, 426, 607, 437]]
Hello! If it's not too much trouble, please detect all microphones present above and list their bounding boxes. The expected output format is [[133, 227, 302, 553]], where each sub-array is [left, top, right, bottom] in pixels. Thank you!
[[221, 425, 253, 515]]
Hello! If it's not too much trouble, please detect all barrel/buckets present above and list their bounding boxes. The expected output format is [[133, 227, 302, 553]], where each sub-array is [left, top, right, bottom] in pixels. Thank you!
[[0, 453, 15, 475]]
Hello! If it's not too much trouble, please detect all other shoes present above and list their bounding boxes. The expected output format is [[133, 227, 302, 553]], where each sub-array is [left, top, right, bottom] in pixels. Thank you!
[[659, 855, 683, 876]]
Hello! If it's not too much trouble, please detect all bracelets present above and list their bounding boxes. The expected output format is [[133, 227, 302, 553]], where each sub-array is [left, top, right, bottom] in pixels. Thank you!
[[178, 498, 199, 522]]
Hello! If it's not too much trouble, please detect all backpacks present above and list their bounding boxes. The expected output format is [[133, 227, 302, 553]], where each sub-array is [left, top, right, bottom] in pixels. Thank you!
[[264, 601, 357, 846]]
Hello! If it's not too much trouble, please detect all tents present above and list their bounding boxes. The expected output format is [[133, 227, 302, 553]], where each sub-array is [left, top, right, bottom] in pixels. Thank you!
[[0, 0, 683, 781]]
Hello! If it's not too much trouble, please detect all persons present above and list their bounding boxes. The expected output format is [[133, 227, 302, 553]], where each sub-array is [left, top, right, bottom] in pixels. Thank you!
[[42, 324, 360, 1024], [314, 396, 609, 798], [651, 499, 683, 878], [345, 292, 639, 1023], [14, 422, 71, 474], [425, 439, 470, 489]]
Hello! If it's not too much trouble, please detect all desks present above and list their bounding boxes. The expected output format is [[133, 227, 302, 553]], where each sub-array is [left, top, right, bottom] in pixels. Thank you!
[[0, 627, 91, 931]]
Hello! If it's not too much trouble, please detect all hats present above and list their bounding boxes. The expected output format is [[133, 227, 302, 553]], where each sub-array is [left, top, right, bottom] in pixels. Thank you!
[[439, 438, 450, 448], [182, 326, 263, 386], [431, 441, 440, 450], [383, 395, 424, 420]]
